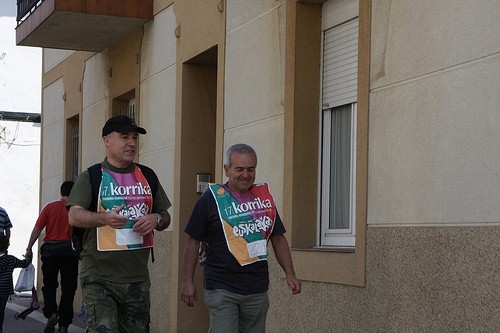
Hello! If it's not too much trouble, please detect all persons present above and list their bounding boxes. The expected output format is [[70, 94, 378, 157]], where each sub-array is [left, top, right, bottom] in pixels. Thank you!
[[0, 236, 32, 333], [180, 144, 302, 333], [0, 207, 12, 237], [64, 115, 172, 333], [27, 180, 79, 333]]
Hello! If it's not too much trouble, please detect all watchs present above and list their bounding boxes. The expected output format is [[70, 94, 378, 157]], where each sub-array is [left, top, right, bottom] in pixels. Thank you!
[[156, 213, 163, 227]]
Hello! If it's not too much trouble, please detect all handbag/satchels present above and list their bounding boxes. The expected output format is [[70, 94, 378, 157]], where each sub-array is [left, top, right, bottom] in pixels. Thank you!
[[15, 263, 35, 292]]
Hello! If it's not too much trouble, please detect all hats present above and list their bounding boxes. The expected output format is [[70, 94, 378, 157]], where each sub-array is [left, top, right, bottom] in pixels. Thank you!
[[102, 116, 146, 137]]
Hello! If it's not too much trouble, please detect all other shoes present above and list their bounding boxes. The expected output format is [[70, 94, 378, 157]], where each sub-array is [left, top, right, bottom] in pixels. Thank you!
[[45, 313, 58, 333], [59, 326, 68, 333]]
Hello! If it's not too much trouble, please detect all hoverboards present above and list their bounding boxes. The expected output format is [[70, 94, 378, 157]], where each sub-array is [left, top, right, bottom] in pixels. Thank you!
[[13, 254, 40, 321]]
